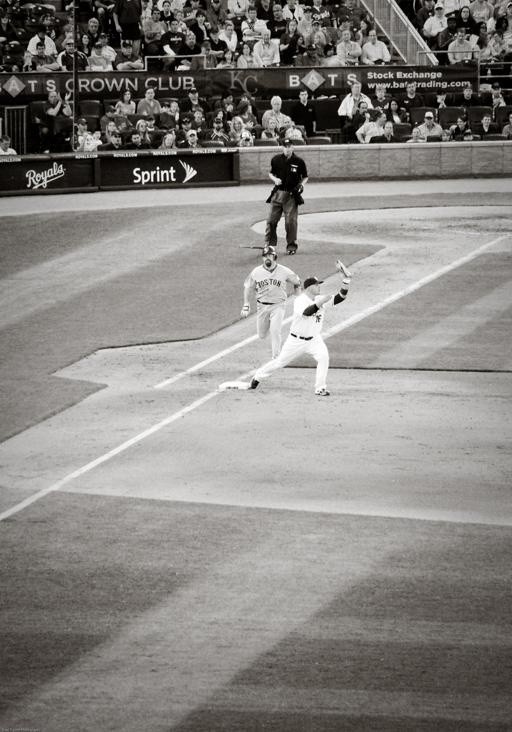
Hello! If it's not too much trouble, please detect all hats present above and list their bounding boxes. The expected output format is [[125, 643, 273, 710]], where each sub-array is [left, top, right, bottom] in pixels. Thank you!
[[262, 245, 276, 256], [282, 138, 292, 145], [304, 276, 324, 288], [37, 2, 512, 137]]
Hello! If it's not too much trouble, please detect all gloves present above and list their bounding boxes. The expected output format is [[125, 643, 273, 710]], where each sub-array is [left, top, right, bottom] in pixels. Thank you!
[[240, 302, 250, 317]]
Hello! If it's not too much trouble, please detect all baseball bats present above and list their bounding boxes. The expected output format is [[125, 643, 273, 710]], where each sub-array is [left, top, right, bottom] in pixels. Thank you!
[[236, 246, 264, 250]]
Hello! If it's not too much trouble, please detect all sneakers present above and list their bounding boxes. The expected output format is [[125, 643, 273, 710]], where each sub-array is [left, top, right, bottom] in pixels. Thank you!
[[249, 376, 262, 392], [316, 388, 329, 396], [286, 243, 298, 255]]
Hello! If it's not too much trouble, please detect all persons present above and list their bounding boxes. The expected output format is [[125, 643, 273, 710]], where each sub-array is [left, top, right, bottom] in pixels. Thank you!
[[251, 259, 352, 395], [239, 246, 301, 360], [264, 138, 310, 256], [0, 1, 512, 156]]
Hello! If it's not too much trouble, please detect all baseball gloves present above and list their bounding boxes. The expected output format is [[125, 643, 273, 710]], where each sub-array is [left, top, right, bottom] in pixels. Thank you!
[[335, 260, 353, 280]]
